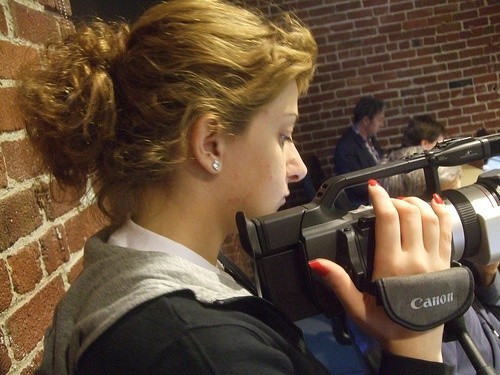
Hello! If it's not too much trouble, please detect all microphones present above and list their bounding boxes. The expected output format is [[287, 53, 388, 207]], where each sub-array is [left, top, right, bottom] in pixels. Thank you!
[[428, 134, 500, 167]]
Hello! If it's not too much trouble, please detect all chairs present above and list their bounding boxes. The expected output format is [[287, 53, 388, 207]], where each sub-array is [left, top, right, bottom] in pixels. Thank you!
[[300, 154, 339, 209]]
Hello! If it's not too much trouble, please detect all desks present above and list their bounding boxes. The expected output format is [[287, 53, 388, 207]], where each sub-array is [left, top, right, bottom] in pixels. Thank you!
[[461, 162, 484, 188]]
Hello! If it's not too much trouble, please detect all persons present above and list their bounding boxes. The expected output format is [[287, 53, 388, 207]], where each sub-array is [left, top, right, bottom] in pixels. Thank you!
[[20, 0, 452, 375], [333, 96, 499, 375]]
